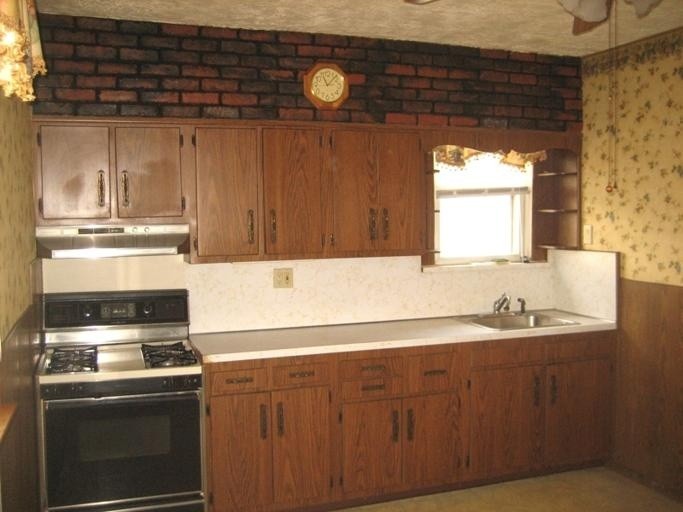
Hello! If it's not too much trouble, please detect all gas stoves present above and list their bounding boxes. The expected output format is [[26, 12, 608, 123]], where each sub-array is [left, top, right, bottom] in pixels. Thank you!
[[40, 339, 200, 400]]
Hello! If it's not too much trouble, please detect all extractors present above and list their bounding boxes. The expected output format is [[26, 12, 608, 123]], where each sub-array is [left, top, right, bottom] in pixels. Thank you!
[[36, 222, 188, 260]]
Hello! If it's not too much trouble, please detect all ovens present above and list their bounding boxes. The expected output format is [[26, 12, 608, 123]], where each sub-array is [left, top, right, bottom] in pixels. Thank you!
[[39, 389, 204, 511]]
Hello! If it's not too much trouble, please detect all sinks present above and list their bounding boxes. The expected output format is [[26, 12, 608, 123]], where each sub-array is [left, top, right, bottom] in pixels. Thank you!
[[451, 311, 583, 331]]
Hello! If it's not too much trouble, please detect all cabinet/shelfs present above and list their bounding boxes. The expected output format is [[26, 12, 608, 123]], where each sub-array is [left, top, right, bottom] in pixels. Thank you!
[[335, 343, 467, 506], [426, 169, 440, 254], [322, 122, 427, 257], [463, 329, 617, 485], [204, 353, 335, 511], [32, 116, 183, 226], [535, 172, 578, 250], [183, 119, 328, 264]]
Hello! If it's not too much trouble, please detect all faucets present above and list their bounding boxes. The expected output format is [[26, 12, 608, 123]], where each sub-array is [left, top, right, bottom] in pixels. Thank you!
[[494, 292, 511, 314], [517, 297, 526, 314]]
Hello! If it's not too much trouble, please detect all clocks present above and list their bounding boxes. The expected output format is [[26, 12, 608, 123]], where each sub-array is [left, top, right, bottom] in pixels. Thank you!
[[302, 61, 349, 113]]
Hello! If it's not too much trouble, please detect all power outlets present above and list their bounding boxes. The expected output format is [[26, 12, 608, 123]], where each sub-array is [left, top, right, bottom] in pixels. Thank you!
[[272, 268, 292, 289]]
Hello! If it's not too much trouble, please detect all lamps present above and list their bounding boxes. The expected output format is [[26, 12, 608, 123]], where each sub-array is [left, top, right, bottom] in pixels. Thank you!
[[554, 1, 662, 194]]
